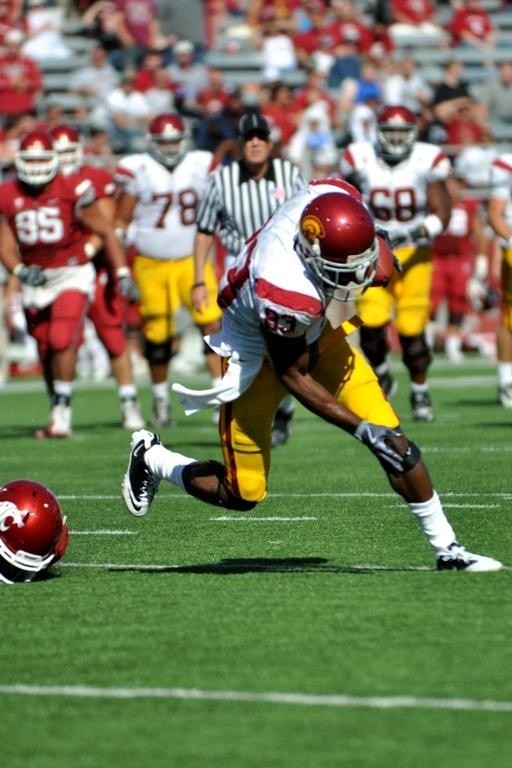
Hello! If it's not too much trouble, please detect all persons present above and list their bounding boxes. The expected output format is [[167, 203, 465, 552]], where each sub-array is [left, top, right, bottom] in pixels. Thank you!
[[122, 178, 506, 572], [1, 0, 512, 441]]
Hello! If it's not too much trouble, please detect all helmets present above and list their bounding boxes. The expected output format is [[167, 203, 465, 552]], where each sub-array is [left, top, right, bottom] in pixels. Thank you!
[[293, 192, 378, 301], [151, 111, 186, 164], [374, 106, 418, 161], [17, 126, 84, 187]]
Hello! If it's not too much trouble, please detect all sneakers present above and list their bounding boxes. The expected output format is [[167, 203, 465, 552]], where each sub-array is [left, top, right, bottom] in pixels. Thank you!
[[121, 428, 161, 518], [270, 408, 291, 447], [380, 373, 434, 424], [495, 382, 512, 410], [435, 549, 502, 573], [45, 396, 76, 437], [121, 397, 173, 432]]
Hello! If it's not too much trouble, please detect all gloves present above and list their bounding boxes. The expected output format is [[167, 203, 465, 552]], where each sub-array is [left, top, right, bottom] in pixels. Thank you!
[[391, 222, 431, 251], [17, 264, 49, 288], [356, 421, 405, 472], [117, 270, 140, 306]]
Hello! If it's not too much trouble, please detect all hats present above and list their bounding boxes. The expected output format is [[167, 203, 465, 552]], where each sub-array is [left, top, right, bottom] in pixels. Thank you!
[[238, 112, 272, 141]]
